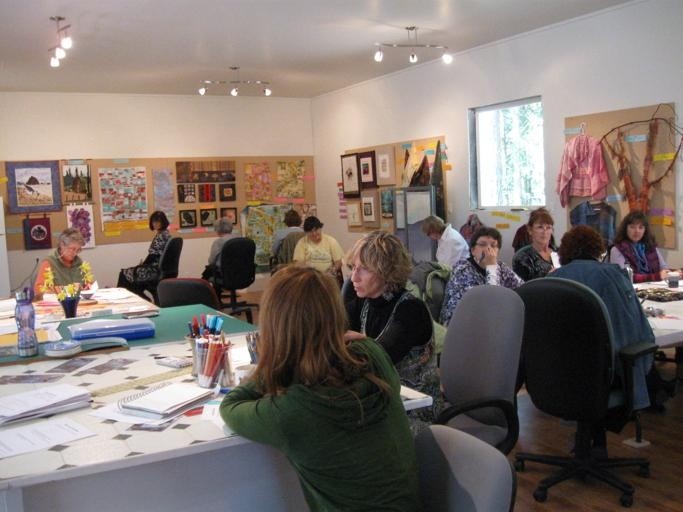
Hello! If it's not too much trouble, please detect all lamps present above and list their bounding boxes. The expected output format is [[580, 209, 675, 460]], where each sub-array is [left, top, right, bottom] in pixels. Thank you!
[[55, 48, 66, 58], [230, 85, 241, 97], [197, 84, 207, 96], [410, 48, 418, 62], [61, 36, 72, 50], [441, 49, 452, 63], [374, 46, 384, 62], [261, 84, 270, 96], [49, 56, 59, 68]]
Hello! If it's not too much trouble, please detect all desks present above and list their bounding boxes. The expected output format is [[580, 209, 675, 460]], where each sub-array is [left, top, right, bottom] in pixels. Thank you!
[[631, 277, 683, 349], [0, 286, 158, 347], [0, 303, 435, 511]]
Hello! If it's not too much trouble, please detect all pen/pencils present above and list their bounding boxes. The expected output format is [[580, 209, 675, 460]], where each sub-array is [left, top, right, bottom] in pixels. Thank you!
[[245, 333, 261, 364], [194, 337, 234, 388], [187, 313, 224, 337], [478, 252, 484, 263]]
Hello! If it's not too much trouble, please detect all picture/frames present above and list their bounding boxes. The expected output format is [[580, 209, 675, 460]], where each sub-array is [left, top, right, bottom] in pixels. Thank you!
[[5, 160, 63, 213], [178, 209, 197, 228], [358, 150, 377, 188], [345, 198, 363, 233], [220, 207, 237, 224], [340, 152, 361, 198], [200, 208, 217, 227], [360, 191, 381, 229], [375, 146, 396, 185]]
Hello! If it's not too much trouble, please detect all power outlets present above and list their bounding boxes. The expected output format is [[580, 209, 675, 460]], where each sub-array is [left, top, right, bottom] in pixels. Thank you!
[[34, 254, 41, 263]]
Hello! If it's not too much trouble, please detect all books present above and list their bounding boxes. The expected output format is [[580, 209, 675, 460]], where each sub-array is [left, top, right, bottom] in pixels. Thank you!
[[118, 380, 215, 420]]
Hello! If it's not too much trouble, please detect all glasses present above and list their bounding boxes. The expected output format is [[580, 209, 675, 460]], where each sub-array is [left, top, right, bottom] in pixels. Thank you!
[[349, 262, 378, 274], [530, 224, 554, 232], [62, 245, 82, 254], [475, 241, 499, 249]]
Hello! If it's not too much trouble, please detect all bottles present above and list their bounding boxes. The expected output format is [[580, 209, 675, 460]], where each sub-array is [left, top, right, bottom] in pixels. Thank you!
[[623, 262, 634, 284], [15, 291, 38, 358]]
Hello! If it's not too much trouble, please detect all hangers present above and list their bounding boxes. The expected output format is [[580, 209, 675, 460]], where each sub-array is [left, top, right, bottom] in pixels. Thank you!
[[580, 121, 588, 144], [588, 196, 604, 211]]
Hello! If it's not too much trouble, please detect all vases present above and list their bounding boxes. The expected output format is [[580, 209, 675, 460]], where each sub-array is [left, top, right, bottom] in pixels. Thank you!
[[58, 298, 80, 317]]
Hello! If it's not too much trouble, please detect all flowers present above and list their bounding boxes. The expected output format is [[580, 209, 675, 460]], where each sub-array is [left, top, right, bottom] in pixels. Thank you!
[[39, 259, 94, 301]]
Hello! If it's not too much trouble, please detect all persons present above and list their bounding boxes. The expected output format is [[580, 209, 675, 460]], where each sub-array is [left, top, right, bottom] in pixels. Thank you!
[[546, 226, 656, 407], [220, 264, 428, 512], [273, 210, 345, 274], [438, 228, 525, 325], [423, 215, 470, 267], [343, 229, 446, 423], [513, 208, 561, 282], [208, 217, 237, 288], [609, 210, 683, 283], [34, 228, 94, 300], [116, 211, 173, 296]]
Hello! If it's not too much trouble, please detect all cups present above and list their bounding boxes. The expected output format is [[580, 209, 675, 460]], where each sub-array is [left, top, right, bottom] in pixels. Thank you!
[[184, 332, 225, 376], [196, 345, 234, 388], [665, 272, 680, 288]]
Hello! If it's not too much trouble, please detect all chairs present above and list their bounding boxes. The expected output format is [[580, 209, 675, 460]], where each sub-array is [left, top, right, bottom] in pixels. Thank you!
[[268, 231, 305, 269], [157, 278, 253, 324], [431, 283, 525, 456], [209, 236, 255, 307], [133, 236, 184, 305], [341, 272, 361, 332], [509, 274, 650, 507], [416, 423, 517, 512]]
